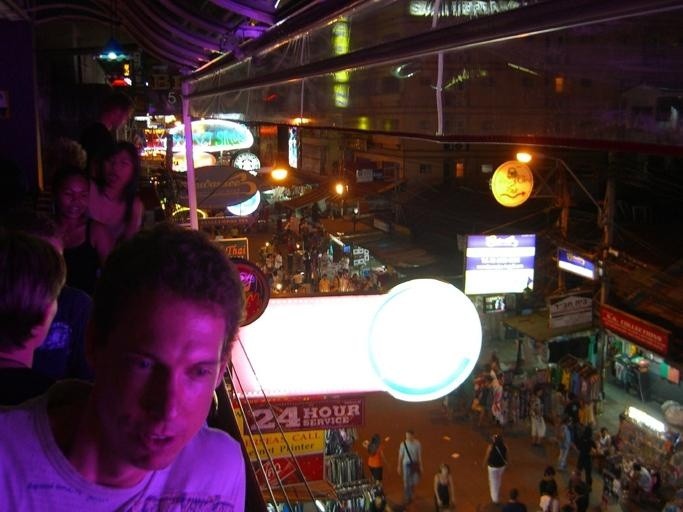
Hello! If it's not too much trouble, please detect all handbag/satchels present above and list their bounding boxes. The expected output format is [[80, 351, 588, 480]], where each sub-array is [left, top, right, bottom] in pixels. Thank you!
[[408, 462, 421, 474]]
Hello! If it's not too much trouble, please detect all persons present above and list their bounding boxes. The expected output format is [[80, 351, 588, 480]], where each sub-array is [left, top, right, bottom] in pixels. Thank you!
[[5, 209, 90, 382], [30, 163, 113, 294], [0, 219, 251, 512], [83, 139, 145, 244], [222, 196, 682, 510], [0, 229, 66, 409]]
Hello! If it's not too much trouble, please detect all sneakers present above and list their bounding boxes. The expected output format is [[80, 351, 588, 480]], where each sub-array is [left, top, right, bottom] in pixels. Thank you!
[[405, 496, 415, 504]]
